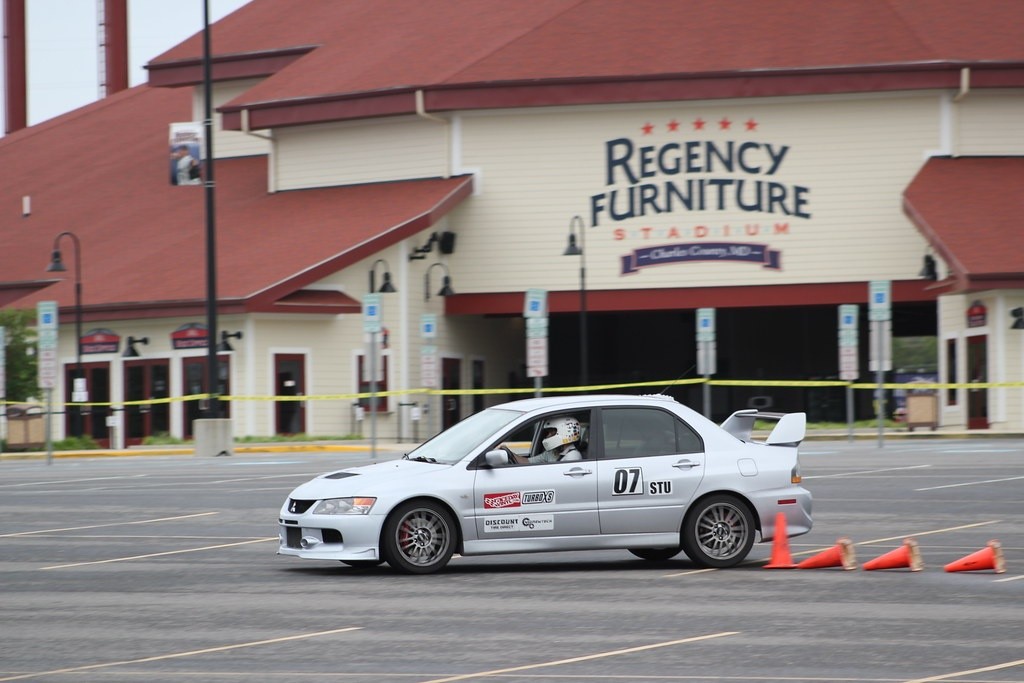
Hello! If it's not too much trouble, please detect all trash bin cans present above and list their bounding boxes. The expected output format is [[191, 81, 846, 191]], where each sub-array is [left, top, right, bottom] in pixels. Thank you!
[[904, 381, 939, 432], [5, 402, 47, 451]]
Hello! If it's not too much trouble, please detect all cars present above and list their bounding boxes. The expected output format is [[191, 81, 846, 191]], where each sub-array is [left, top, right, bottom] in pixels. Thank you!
[[276, 393, 814, 575]]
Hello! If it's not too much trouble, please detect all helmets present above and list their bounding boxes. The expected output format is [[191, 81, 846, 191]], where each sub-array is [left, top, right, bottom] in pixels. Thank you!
[[541, 414, 581, 452]]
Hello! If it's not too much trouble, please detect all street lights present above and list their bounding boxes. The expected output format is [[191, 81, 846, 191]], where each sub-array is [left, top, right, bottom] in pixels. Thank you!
[[45, 229, 86, 439], [561, 215, 590, 389]]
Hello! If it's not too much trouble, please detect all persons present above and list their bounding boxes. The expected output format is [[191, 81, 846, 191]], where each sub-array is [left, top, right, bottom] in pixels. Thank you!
[[497, 414, 583, 463]]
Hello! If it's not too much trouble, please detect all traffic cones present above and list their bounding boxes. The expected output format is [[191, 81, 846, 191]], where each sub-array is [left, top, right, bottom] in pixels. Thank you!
[[795, 537, 856, 571], [761, 511, 800, 569], [943, 539, 1010, 575], [861, 537, 926, 573]]
